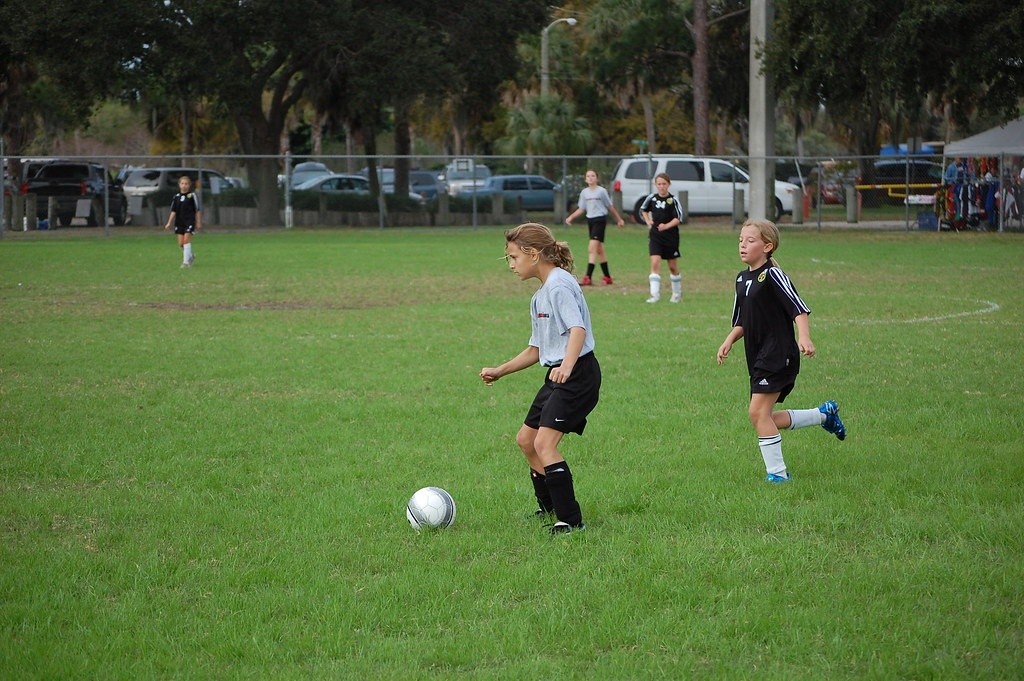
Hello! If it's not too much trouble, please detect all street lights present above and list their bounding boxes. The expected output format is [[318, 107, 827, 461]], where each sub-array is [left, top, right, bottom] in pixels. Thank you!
[[540, 18, 579, 101]]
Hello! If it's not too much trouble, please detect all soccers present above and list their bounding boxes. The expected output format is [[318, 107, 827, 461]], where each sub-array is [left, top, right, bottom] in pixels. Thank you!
[[406, 486, 458, 531]]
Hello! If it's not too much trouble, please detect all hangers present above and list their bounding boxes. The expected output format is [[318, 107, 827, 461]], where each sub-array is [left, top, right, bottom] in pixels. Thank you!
[[943, 174, 1014, 186]]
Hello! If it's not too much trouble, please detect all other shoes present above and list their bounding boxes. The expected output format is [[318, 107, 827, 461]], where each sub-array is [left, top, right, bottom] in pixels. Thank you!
[[669, 293, 682, 302], [600, 276, 612, 286], [526, 509, 555, 518], [541, 522, 586, 537], [646, 293, 660, 303], [579, 275, 590, 285], [188, 254, 196, 264], [181, 263, 191, 268]]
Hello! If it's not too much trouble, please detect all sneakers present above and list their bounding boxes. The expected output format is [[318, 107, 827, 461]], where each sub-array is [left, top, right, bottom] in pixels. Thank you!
[[819, 400, 847, 441], [766, 473, 791, 484]]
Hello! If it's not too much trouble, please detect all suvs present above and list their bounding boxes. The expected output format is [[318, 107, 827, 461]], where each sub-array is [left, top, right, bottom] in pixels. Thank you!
[[610, 153, 802, 224]]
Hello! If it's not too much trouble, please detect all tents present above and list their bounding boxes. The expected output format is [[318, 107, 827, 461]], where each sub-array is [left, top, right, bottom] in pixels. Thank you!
[[940, 114, 1024, 233]]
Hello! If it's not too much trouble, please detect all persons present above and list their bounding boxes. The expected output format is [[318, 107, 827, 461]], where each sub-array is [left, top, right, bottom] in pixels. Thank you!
[[985, 167, 998, 181], [166, 176, 202, 268], [479, 224, 603, 540], [945, 157, 968, 186], [641, 173, 683, 304], [566, 170, 624, 286], [716, 219, 846, 483]]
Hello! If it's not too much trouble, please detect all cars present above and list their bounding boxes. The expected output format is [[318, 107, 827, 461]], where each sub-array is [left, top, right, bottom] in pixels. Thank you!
[[123, 166, 260, 212], [206, 156, 493, 212], [814, 160, 949, 209], [21, 159, 128, 226], [456, 173, 571, 214]]
